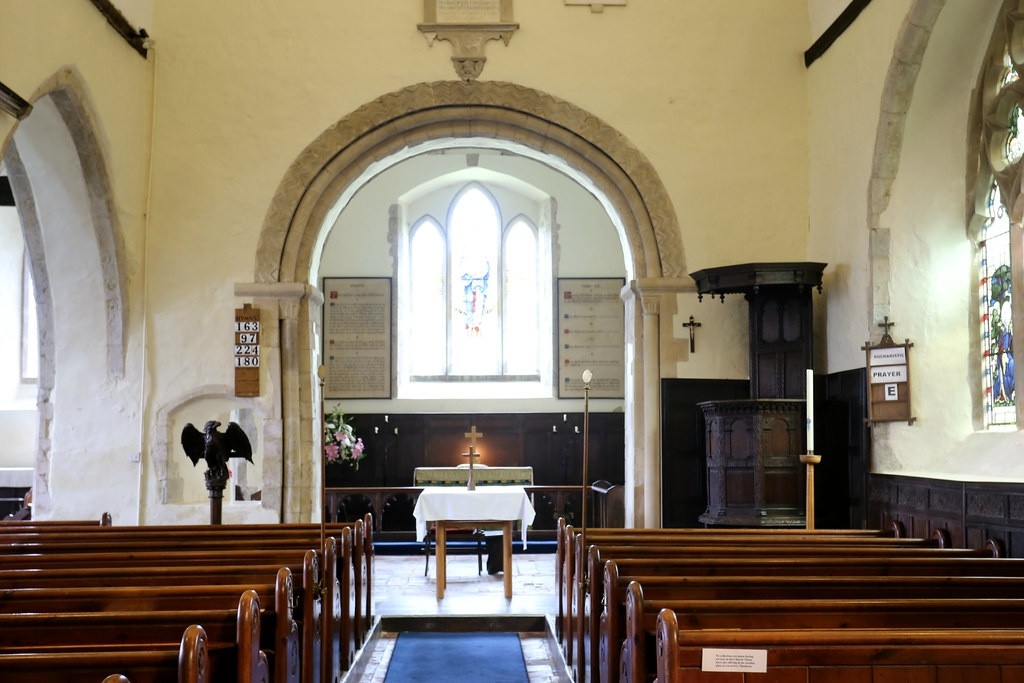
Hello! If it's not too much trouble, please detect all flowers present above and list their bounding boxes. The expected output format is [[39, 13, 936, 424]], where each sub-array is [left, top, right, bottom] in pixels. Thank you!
[[324, 402, 367, 470]]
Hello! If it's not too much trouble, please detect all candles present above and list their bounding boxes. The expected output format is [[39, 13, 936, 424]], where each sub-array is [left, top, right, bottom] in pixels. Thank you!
[[807, 369, 813, 450]]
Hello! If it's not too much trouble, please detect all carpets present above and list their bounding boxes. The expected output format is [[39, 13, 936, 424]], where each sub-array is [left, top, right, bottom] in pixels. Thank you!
[[382, 632, 530, 683]]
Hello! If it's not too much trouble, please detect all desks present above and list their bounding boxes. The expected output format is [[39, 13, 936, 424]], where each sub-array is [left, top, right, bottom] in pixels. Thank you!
[[413, 466, 537, 600]]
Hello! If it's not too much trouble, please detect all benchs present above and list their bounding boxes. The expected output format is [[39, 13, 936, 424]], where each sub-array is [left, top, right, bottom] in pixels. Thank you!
[[554, 515, 1024, 683], [0, 512, 374, 683]]
